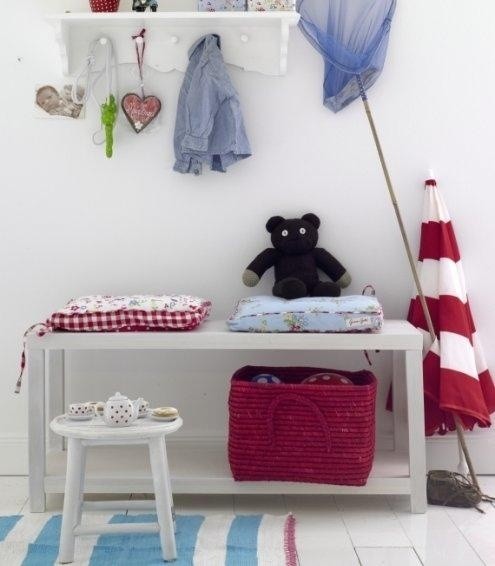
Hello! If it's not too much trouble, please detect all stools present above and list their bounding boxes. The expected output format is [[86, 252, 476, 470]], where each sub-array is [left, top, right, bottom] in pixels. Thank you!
[[49, 408, 182, 563]]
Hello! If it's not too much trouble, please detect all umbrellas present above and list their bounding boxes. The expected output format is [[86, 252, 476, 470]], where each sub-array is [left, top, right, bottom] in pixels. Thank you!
[[385, 169, 495, 484]]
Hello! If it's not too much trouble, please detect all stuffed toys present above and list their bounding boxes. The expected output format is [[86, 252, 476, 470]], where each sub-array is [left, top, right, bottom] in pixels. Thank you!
[[242, 213, 352, 300]]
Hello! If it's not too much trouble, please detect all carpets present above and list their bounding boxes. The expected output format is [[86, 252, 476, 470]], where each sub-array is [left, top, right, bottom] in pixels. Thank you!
[[1, 515, 297, 566]]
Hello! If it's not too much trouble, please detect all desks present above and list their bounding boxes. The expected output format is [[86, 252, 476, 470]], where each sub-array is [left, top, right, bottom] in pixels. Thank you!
[[25, 311, 427, 514]]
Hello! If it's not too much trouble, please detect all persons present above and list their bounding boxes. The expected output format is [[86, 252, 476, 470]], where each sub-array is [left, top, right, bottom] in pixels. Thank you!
[[36, 86, 80, 118]]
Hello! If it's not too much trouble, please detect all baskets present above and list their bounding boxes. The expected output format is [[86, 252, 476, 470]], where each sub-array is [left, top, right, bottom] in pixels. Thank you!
[[227, 366, 377, 485]]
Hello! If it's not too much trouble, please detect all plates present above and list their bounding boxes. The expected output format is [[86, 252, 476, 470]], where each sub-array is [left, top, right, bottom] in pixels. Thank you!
[[138, 413, 150, 417], [150, 415, 180, 421], [67, 416, 95, 421]]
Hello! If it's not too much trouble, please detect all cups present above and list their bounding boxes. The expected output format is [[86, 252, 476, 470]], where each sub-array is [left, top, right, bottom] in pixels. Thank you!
[[69, 404, 95, 416], [138, 400, 149, 412]]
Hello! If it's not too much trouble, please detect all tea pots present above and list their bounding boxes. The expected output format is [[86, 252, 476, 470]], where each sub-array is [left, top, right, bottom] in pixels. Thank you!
[[96, 392, 143, 427]]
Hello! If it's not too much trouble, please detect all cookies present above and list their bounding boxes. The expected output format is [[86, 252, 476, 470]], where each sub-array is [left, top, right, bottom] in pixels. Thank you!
[[150, 407, 178, 417]]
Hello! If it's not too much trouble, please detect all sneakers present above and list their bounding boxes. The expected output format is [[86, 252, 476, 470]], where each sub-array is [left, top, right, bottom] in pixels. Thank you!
[[427, 469, 482, 507]]
